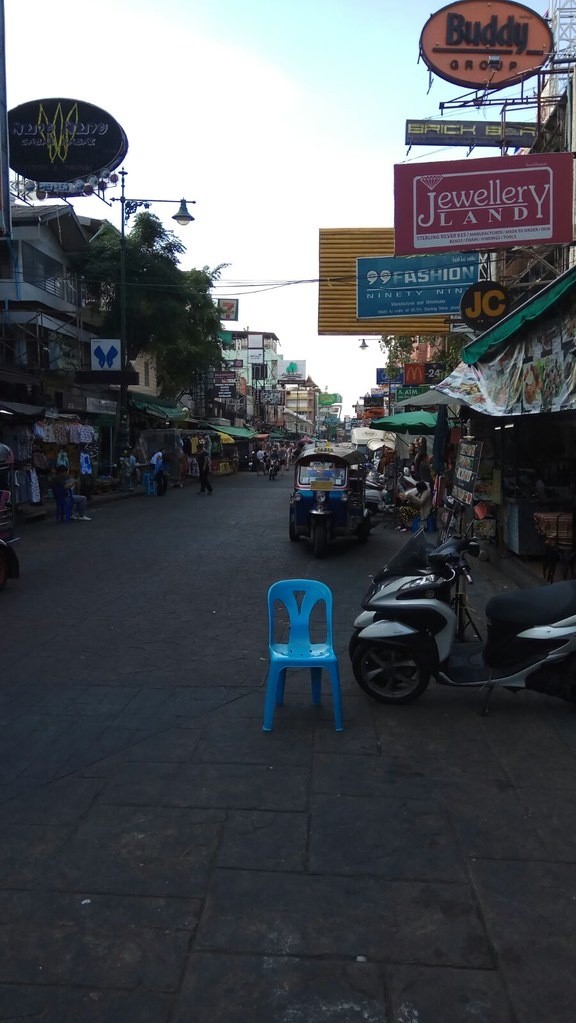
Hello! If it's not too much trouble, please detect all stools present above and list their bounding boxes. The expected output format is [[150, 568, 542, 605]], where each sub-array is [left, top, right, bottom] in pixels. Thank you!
[[412, 515, 433, 534], [142, 471, 158, 497]]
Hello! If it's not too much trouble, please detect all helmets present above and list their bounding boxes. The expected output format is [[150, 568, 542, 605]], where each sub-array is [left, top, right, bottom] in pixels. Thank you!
[[272, 448, 277, 451]]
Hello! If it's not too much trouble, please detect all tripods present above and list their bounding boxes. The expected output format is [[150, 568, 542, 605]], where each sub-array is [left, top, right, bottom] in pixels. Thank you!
[[448, 499, 483, 643]]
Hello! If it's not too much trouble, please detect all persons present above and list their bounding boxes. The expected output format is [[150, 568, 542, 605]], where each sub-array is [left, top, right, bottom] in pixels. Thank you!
[[51, 465, 92, 522], [279, 444, 295, 476], [130, 449, 140, 486], [266, 448, 280, 461], [195, 444, 215, 495], [395, 482, 432, 532], [151, 457, 168, 496], [150, 448, 166, 471], [257, 444, 268, 475]]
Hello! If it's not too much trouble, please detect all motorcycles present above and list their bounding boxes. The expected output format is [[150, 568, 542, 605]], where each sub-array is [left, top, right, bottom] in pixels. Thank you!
[[267, 458, 278, 480], [348, 526, 576, 716], [366, 468, 421, 516], [289, 446, 368, 559]]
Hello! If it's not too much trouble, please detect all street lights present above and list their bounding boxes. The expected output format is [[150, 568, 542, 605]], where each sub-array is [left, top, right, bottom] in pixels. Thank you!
[[116, 196, 196, 491]]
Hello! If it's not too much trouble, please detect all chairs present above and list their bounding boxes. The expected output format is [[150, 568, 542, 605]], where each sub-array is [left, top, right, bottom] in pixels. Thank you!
[[262, 579, 344, 734], [51, 484, 77, 523]]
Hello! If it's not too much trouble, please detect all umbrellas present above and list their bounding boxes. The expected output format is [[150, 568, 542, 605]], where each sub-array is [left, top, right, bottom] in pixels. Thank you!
[[300, 435, 319, 442], [369, 409, 454, 435], [395, 389, 465, 405]]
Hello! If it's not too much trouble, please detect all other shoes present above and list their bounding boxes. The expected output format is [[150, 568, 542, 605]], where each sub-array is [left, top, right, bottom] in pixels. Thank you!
[[195, 491, 205, 496], [281, 474, 283, 476], [400, 528, 408, 532], [70, 514, 77, 520], [79, 515, 92, 521], [207, 490, 215, 496], [286, 469, 289, 471], [395, 525, 403, 530]]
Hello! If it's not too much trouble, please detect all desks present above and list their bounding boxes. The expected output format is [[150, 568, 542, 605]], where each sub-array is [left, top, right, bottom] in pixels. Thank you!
[[534, 512, 573, 584], [505, 503, 573, 561]]
